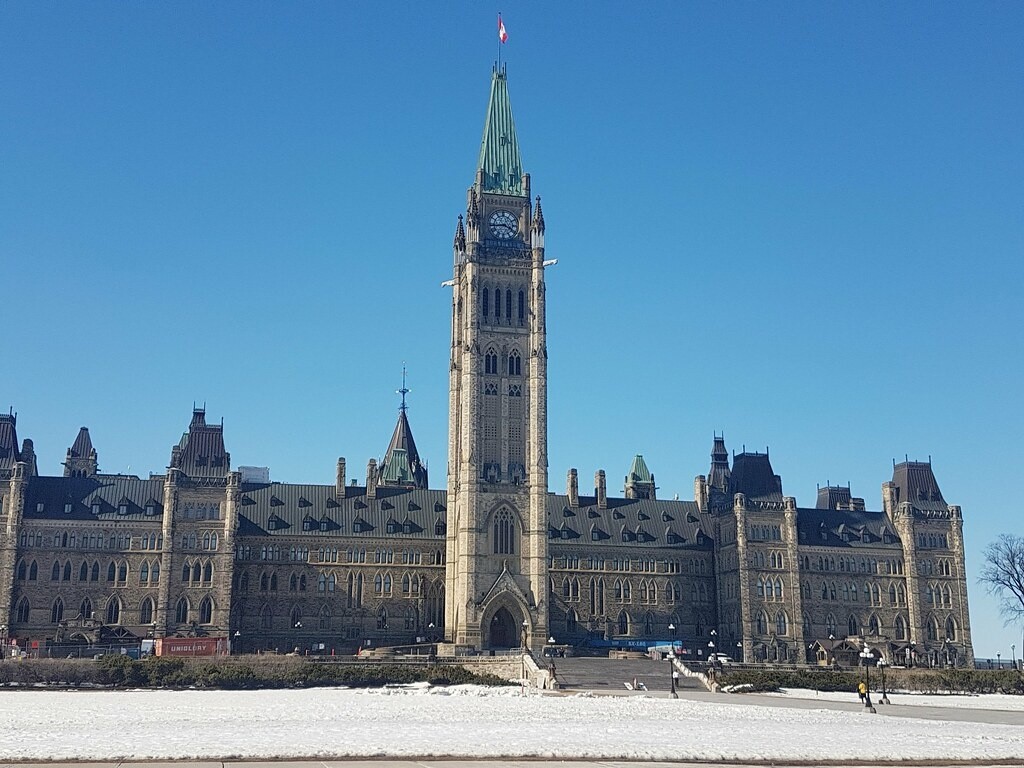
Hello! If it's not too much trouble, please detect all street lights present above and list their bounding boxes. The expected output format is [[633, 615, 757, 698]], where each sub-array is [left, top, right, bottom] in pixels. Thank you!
[[737, 641, 742, 663], [708, 642, 715, 656], [234, 631, 241, 658], [997, 651, 1001, 670], [1011, 644, 1015, 666], [295, 622, 302, 648], [808, 644, 814, 664], [829, 634, 835, 658], [877, 657, 887, 699], [429, 623, 435, 654], [860, 648, 872, 707], [669, 624, 675, 652], [548, 637, 555, 667], [711, 630, 718, 660], [666, 650, 678, 699], [0, 625, 7, 660], [151, 621, 158, 654]]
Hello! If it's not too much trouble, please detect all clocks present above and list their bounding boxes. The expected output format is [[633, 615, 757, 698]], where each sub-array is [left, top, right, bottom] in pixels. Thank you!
[[487, 209, 521, 240]]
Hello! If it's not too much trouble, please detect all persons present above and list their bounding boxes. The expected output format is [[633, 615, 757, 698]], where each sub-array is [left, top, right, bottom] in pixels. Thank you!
[[858, 679, 866, 704], [673, 668, 679, 688]]
